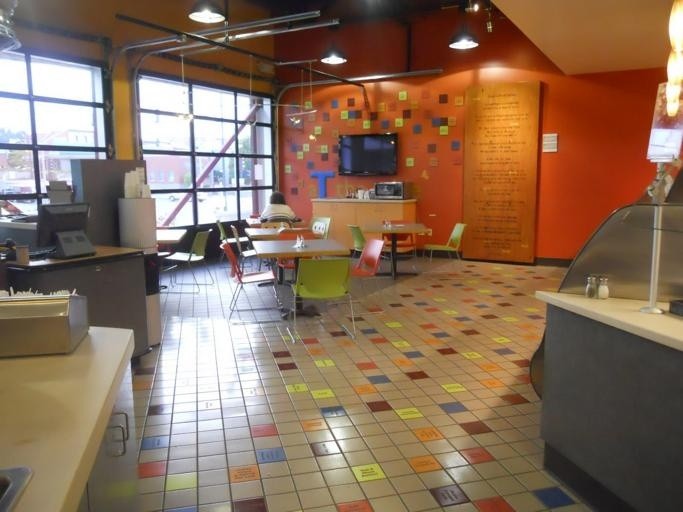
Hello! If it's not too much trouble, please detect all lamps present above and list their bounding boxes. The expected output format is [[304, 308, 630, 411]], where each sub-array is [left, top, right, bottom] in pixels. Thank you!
[[450, 7, 478, 51], [186, 0, 226, 23], [321, 26, 346, 66]]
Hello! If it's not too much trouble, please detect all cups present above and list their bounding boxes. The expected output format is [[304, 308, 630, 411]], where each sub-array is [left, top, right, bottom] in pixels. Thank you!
[[586, 275, 597, 298], [357, 190, 363, 198], [599, 277, 609, 298]]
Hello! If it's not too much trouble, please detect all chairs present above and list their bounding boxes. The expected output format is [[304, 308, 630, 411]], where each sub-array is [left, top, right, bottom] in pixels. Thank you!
[[219, 242, 283, 320], [348, 224, 367, 261], [287, 258, 356, 343], [164, 229, 215, 294], [309, 216, 330, 238], [230, 225, 270, 274], [422, 223, 466, 264], [216, 221, 249, 267], [350, 239, 385, 299]]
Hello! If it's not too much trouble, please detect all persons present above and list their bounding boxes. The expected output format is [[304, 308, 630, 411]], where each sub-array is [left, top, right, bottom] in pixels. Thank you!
[[260, 192, 301, 223]]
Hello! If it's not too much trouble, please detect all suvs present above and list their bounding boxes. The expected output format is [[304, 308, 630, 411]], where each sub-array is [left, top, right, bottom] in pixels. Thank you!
[[1, 186, 35, 204]]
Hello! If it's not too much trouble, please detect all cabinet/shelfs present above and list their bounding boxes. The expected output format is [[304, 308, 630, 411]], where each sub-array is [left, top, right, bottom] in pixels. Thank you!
[[1, 327, 139, 512], [534, 204, 683, 512], [309, 199, 417, 253]]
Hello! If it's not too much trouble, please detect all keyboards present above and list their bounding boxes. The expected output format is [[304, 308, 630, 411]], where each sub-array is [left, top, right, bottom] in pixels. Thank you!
[[28, 249, 50, 258]]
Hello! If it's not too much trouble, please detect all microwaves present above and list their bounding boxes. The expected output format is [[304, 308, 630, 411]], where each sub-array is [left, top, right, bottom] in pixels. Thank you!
[[375, 182, 405, 199]]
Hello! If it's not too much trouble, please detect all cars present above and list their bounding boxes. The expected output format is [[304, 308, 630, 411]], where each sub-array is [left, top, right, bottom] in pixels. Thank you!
[[166, 192, 207, 203]]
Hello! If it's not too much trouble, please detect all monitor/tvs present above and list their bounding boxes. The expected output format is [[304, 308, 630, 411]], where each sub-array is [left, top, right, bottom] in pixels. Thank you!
[[338, 133, 399, 176], [37, 202, 97, 261]]
